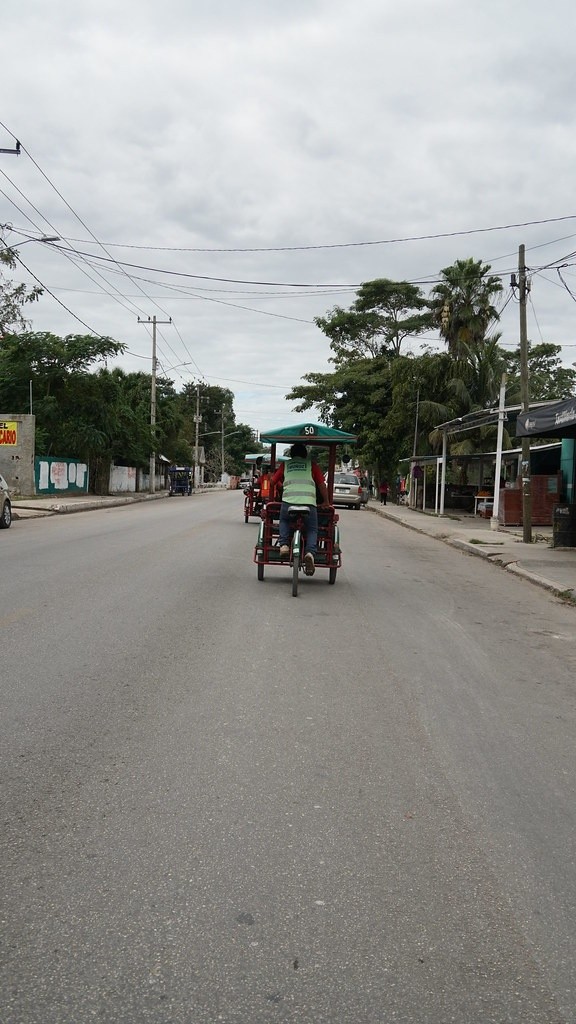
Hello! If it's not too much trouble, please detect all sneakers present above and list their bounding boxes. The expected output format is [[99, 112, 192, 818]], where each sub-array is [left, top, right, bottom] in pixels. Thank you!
[[304, 552, 315, 576], [279, 545, 289, 558]]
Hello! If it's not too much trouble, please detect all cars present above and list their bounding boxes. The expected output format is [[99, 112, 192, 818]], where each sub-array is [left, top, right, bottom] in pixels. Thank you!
[[0, 474, 12, 529], [238, 478, 251, 488]]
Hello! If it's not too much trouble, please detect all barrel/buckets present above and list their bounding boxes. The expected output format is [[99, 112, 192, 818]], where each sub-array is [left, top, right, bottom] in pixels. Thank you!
[[490, 519, 499, 531]]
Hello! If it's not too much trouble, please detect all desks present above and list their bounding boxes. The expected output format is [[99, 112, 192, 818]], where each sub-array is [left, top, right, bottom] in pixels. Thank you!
[[475, 496, 494, 515]]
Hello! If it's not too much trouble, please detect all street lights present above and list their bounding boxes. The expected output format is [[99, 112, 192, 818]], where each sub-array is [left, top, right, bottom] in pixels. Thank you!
[[0, 234, 60, 253], [150, 362, 193, 493], [221, 431, 242, 487], [193, 431, 223, 492]]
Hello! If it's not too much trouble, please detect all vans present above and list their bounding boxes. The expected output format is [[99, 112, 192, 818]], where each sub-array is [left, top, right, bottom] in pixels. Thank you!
[[325, 470, 369, 510]]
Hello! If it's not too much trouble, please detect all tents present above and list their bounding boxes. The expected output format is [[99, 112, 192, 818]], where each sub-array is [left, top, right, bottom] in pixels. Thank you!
[[515, 396, 576, 439]]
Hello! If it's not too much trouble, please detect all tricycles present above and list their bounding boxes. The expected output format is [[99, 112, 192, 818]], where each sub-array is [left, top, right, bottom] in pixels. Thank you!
[[170, 465, 194, 497], [255, 423, 358, 597], [242, 453, 293, 523]]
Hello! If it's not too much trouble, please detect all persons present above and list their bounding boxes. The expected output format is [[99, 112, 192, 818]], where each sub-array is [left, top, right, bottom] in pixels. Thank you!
[[380, 481, 387, 506], [369, 482, 374, 499], [257, 444, 328, 576]]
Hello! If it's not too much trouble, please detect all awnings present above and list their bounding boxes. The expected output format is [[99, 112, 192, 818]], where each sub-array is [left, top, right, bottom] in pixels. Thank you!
[[433, 399, 561, 430]]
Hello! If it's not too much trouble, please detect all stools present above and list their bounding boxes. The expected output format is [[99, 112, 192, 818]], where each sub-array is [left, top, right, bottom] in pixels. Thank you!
[[478, 503, 493, 519]]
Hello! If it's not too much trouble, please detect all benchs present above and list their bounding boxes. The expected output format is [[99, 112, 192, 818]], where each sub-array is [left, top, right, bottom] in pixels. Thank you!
[[264, 500, 336, 527], [453, 497, 474, 510]]
